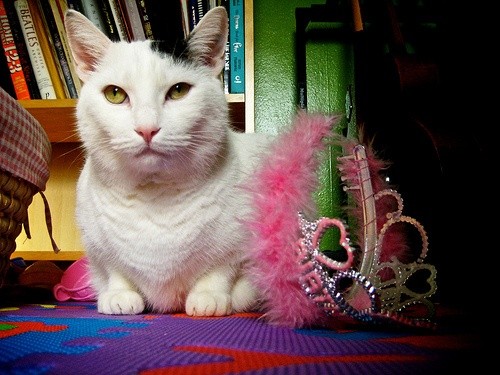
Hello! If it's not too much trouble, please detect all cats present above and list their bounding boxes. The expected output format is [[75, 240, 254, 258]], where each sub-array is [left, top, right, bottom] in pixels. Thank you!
[[62, 5, 273, 319]]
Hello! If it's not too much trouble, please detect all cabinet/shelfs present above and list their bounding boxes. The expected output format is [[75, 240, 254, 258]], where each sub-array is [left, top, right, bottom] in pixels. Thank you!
[[0, 0, 257, 271]]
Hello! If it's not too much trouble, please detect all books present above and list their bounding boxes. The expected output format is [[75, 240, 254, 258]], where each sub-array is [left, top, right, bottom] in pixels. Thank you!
[[1, 0, 246, 99]]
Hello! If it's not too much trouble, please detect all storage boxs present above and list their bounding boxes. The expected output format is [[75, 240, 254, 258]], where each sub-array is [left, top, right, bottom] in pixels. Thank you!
[[0, 90, 53, 266]]
